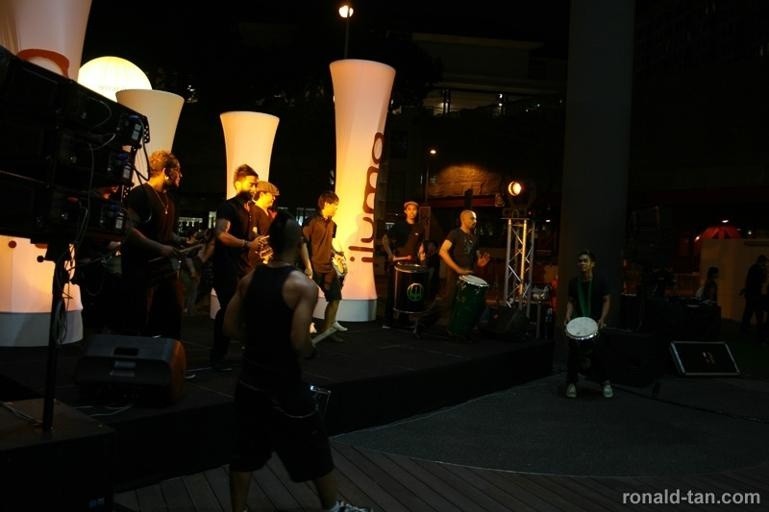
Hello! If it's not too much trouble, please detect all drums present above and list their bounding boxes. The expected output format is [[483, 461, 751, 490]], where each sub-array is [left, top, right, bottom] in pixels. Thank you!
[[249, 241, 275, 273], [563, 317, 607, 369], [446, 273, 490, 334], [391, 261, 431, 317]]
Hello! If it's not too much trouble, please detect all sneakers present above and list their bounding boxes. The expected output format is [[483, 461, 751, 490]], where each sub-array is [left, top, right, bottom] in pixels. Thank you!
[[210, 357, 233, 372], [566, 382, 577, 398], [330, 321, 348, 332], [600, 384, 614, 398], [322, 500, 374, 512], [322, 333, 344, 344]]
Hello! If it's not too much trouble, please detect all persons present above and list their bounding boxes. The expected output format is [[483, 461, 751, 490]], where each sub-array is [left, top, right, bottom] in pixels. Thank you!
[[115, 154, 492, 511], [739, 254, 768, 334], [703, 267, 718, 301], [563, 248, 615, 399]]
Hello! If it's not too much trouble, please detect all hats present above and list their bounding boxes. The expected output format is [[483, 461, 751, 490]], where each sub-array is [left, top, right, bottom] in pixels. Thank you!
[[256, 181, 280, 195]]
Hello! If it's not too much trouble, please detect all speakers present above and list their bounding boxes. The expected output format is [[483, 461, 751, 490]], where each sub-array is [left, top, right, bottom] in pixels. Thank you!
[[496, 304, 529, 344], [73, 334, 187, 408]]
